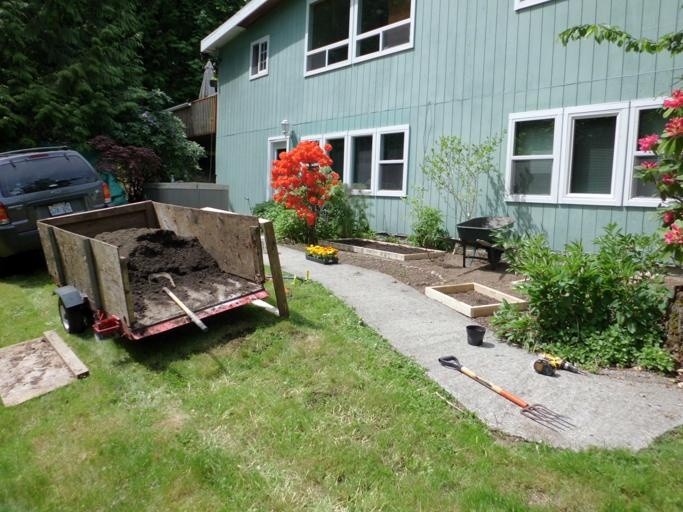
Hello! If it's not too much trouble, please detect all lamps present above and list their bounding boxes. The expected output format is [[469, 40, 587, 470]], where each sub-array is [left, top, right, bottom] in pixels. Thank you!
[[280, 119, 289, 138]]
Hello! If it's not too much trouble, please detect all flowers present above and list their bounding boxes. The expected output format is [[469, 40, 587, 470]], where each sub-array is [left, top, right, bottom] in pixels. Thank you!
[[306, 243, 338, 259]]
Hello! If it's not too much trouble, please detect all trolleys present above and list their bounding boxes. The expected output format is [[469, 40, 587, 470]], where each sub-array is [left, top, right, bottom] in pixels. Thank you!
[[434, 214, 519, 266]]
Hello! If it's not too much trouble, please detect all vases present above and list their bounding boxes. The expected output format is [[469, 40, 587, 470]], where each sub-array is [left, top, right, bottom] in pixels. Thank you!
[[307, 254, 338, 265], [466, 325, 486, 346]]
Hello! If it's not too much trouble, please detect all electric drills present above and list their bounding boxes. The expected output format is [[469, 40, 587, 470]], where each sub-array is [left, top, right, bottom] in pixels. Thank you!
[[534, 353, 587, 376]]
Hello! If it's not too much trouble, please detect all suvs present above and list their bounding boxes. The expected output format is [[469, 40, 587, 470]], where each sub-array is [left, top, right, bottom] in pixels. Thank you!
[[0, 141, 112, 265]]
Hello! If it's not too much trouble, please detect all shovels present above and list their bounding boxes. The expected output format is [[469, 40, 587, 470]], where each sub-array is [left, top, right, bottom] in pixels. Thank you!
[[148, 271, 209, 330]]
